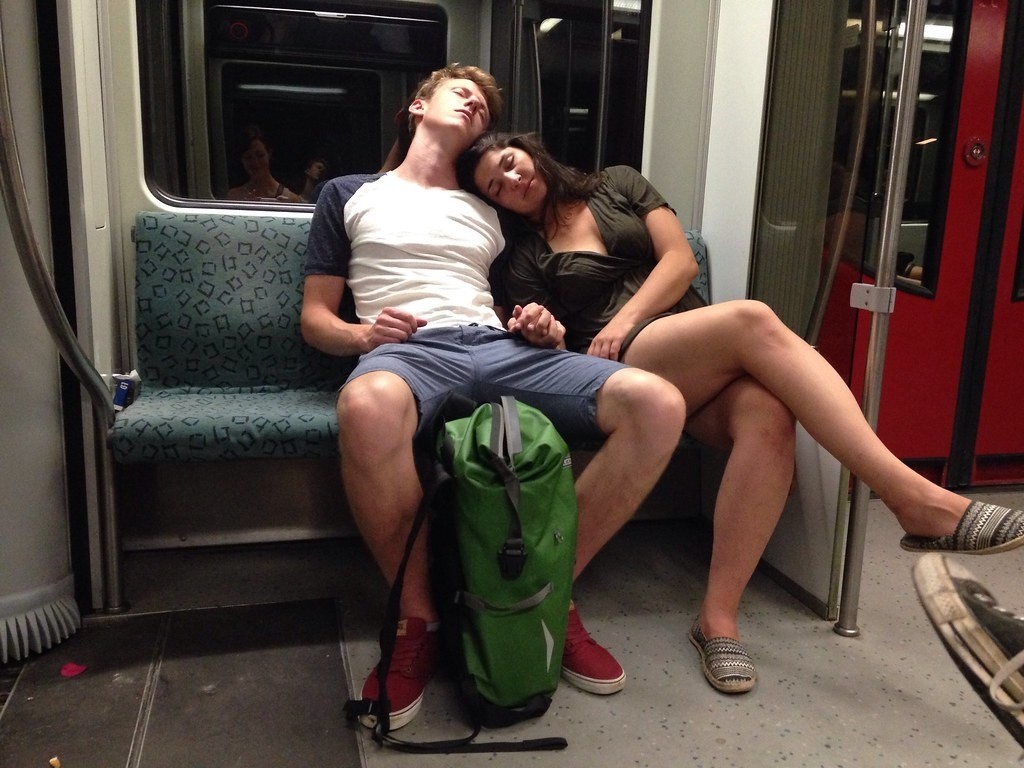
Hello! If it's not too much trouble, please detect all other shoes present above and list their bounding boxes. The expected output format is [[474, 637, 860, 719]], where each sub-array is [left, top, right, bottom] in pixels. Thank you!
[[690, 616, 757, 696], [898, 499, 1024, 556]]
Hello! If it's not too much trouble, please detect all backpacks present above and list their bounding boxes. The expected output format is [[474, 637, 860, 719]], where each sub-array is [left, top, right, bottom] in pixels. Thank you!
[[374, 396, 578, 752]]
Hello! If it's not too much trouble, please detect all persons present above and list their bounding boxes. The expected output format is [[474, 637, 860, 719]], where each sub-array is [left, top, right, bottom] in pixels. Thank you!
[[223, 133, 298, 204], [294, 157, 329, 201], [297, 60, 684, 730], [896, 251, 924, 280], [825, 152, 865, 260], [455, 131, 1023, 697]]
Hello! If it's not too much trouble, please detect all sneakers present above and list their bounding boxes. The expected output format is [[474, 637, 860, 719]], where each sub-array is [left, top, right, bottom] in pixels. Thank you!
[[359, 616, 443, 730], [914, 555, 1024, 757], [560, 600, 627, 696]]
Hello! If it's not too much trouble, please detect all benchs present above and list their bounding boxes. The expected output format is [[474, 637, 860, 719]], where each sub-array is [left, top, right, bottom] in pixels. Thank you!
[[106, 209, 711, 463]]
